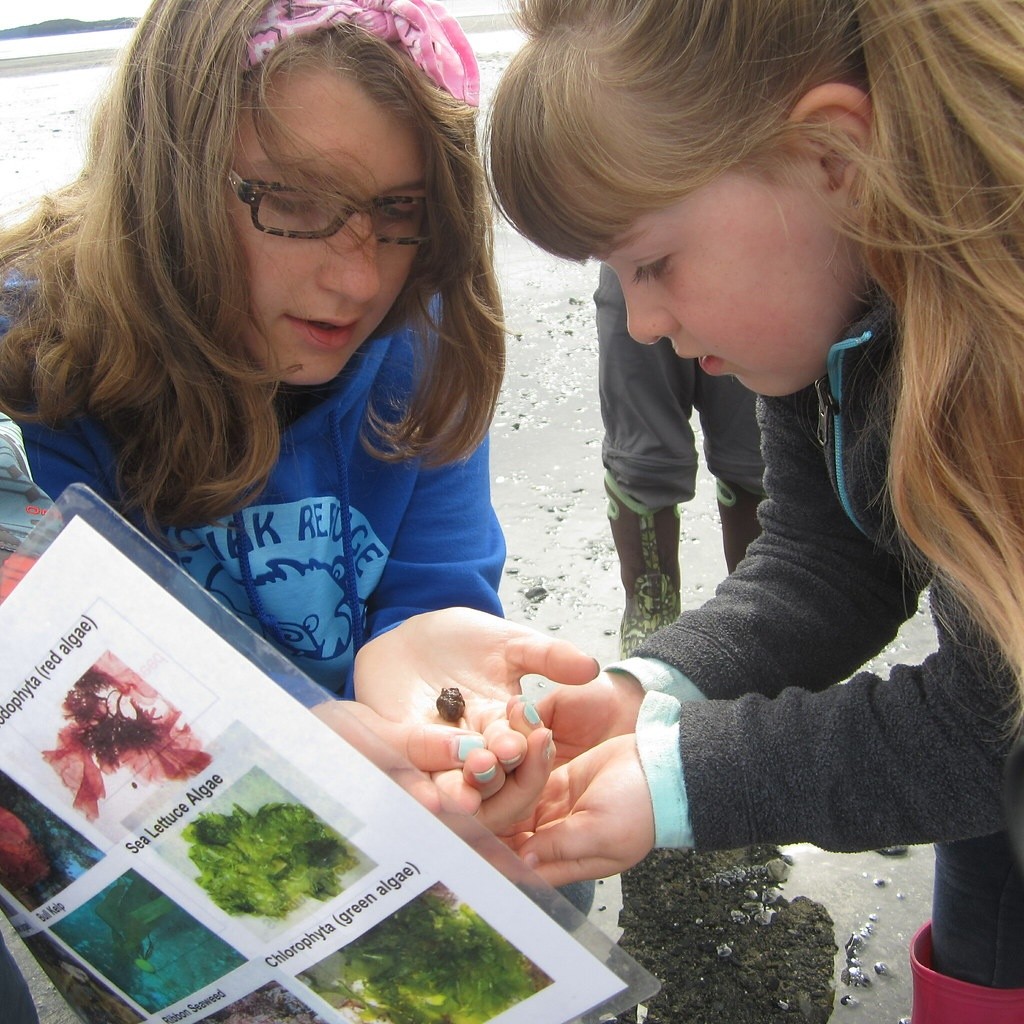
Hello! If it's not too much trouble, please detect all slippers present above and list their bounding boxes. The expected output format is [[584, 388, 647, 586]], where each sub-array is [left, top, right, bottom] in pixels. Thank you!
[[909, 920, 1024, 1024]]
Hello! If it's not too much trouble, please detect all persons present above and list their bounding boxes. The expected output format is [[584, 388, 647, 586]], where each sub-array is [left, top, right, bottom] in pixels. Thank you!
[[480, 0, 1023, 1024], [1, 4, 597, 847], [594, 260, 767, 660]]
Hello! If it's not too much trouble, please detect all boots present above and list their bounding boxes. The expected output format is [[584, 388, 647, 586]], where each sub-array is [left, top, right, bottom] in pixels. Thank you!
[[716, 477, 769, 575], [604, 470, 681, 661]]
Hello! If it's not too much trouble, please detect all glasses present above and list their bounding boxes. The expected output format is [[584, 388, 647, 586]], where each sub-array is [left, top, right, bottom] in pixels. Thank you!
[[227, 167, 432, 246]]
